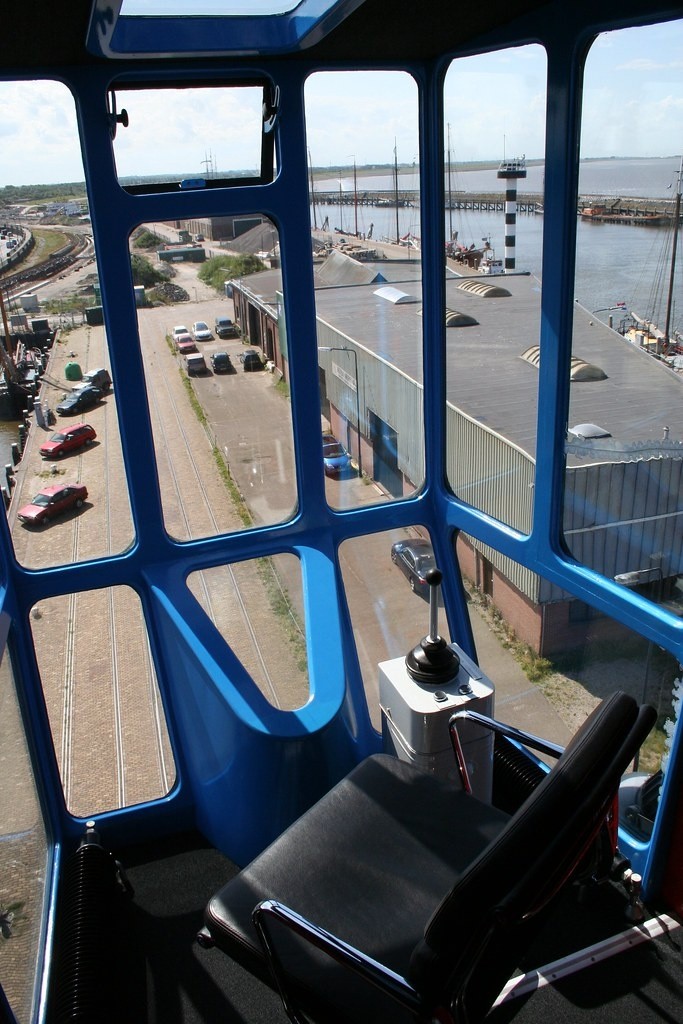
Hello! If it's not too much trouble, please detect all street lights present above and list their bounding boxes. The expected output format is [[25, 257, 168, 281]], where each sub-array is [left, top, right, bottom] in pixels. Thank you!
[[319, 346, 363, 479]]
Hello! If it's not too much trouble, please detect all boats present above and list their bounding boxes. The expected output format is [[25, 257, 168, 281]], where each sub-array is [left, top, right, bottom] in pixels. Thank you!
[[581, 198, 672, 226]]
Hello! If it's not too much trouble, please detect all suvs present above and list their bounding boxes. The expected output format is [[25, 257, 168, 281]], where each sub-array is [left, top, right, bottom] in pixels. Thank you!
[[71, 368, 112, 397], [185, 351, 206, 376], [210, 352, 231, 373], [238, 349, 262, 371], [215, 316, 237, 340]]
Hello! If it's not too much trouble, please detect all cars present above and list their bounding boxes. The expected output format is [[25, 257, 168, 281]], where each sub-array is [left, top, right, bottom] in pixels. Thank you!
[[390, 537, 438, 595], [38, 422, 97, 459], [56, 384, 105, 416], [16, 481, 88, 527], [322, 432, 352, 477], [192, 321, 213, 342], [170, 325, 191, 341], [0, 228, 17, 248], [173, 334, 196, 355], [195, 233, 205, 242]]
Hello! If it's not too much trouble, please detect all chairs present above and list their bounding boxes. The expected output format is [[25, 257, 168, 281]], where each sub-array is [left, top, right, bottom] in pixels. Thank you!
[[196, 691, 658, 1024]]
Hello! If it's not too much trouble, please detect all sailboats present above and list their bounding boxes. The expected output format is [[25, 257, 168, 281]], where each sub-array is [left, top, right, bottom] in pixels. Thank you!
[[592, 150, 682, 377], [308, 120, 530, 275], [0, 256, 43, 419]]
